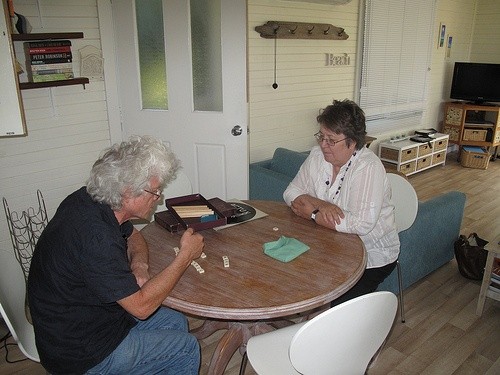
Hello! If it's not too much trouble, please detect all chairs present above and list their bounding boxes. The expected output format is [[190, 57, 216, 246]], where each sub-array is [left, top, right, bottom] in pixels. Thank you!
[[0, 248, 41, 363], [239, 290, 399, 375]]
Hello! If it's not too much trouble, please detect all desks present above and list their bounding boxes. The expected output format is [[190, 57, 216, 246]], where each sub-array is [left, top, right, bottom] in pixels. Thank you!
[[128, 199, 368, 375]]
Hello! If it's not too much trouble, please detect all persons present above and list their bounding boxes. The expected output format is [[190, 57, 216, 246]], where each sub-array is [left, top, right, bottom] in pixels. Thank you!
[[283, 98, 401, 308], [28, 134, 205, 375]]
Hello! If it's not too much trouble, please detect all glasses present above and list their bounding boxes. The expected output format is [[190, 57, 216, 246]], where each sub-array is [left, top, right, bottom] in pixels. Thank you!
[[144, 188, 161, 201], [314, 131, 351, 146]]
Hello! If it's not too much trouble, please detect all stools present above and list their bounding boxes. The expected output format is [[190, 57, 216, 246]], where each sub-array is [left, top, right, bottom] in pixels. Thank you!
[[476, 234, 500, 320]]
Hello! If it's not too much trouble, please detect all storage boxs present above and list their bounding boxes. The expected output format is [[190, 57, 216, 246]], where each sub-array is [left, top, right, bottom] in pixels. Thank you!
[[165, 193, 227, 232]]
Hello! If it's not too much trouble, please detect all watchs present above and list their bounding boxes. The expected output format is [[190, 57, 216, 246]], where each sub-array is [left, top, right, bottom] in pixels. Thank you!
[[311, 209, 319, 222]]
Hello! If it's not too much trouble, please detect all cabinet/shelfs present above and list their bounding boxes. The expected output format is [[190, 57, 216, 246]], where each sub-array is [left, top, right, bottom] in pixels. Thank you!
[[378, 132, 449, 176], [441, 102, 500, 161]]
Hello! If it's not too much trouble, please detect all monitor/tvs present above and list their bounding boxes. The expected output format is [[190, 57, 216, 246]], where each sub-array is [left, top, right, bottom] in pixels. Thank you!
[[450, 61, 500, 107]]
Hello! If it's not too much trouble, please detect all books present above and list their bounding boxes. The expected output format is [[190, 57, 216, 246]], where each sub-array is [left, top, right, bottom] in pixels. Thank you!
[[484, 258, 500, 301], [463, 146, 486, 158], [23, 40, 74, 82]]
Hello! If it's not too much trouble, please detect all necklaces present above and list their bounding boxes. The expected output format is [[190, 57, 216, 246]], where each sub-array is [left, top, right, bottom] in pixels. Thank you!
[[325, 151, 356, 201]]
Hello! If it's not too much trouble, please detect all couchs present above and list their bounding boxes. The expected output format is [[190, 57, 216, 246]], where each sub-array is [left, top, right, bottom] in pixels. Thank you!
[[250, 148, 467, 295]]
